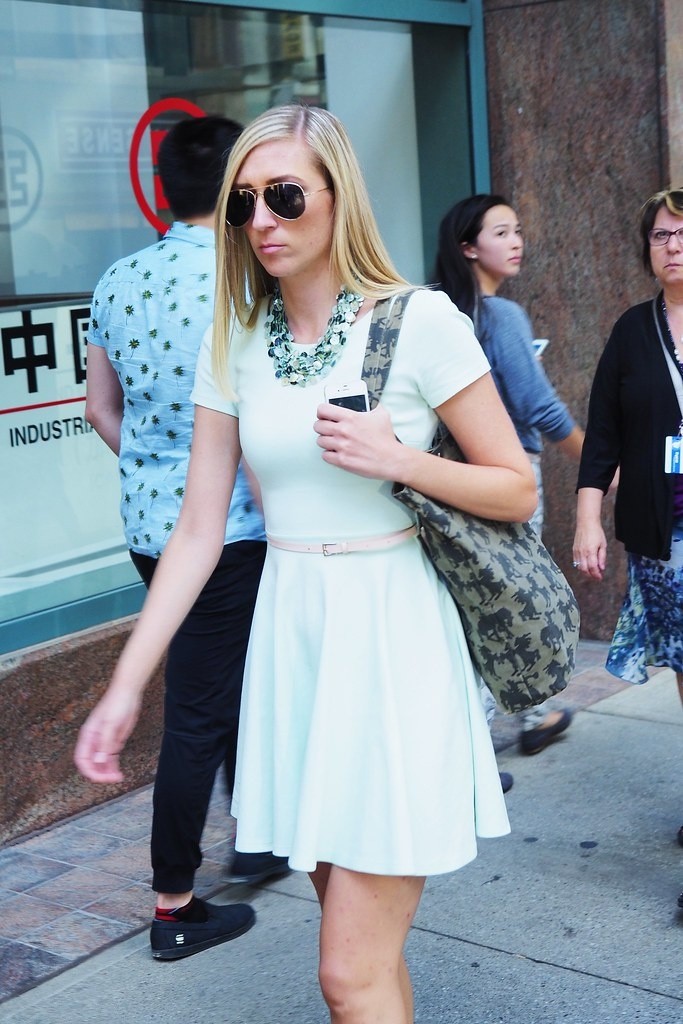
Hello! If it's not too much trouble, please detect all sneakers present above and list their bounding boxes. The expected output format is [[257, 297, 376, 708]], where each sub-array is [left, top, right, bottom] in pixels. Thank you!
[[222, 841, 293, 884], [151, 899, 254, 960]]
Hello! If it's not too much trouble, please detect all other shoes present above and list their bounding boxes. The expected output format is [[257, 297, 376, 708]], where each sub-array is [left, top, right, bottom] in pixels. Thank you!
[[499, 772, 514, 794], [522, 709, 573, 755]]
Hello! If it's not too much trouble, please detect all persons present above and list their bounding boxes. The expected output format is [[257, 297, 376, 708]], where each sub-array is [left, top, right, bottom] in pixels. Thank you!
[[571, 188, 683, 908], [72, 104, 538, 1024], [424, 194, 619, 795], [85, 115, 299, 963]]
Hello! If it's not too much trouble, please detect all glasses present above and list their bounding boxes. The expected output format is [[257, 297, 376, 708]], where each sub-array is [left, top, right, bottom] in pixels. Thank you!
[[647, 226, 683, 246], [226, 182, 333, 229]]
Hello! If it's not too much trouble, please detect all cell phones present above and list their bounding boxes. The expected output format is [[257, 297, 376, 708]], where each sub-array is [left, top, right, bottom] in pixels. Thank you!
[[323, 380, 370, 422], [532, 338, 550, 357]]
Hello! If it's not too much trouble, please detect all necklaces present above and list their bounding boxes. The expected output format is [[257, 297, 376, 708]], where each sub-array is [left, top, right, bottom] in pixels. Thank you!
[[263, 272, 366, 388]]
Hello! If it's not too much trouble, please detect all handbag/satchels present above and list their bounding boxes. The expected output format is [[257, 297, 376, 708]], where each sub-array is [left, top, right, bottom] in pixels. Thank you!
[[360, 287, 581, 713]]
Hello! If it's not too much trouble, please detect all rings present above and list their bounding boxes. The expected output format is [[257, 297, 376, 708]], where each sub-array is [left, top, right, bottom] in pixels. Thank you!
[[572, 561, 580, 568]]
